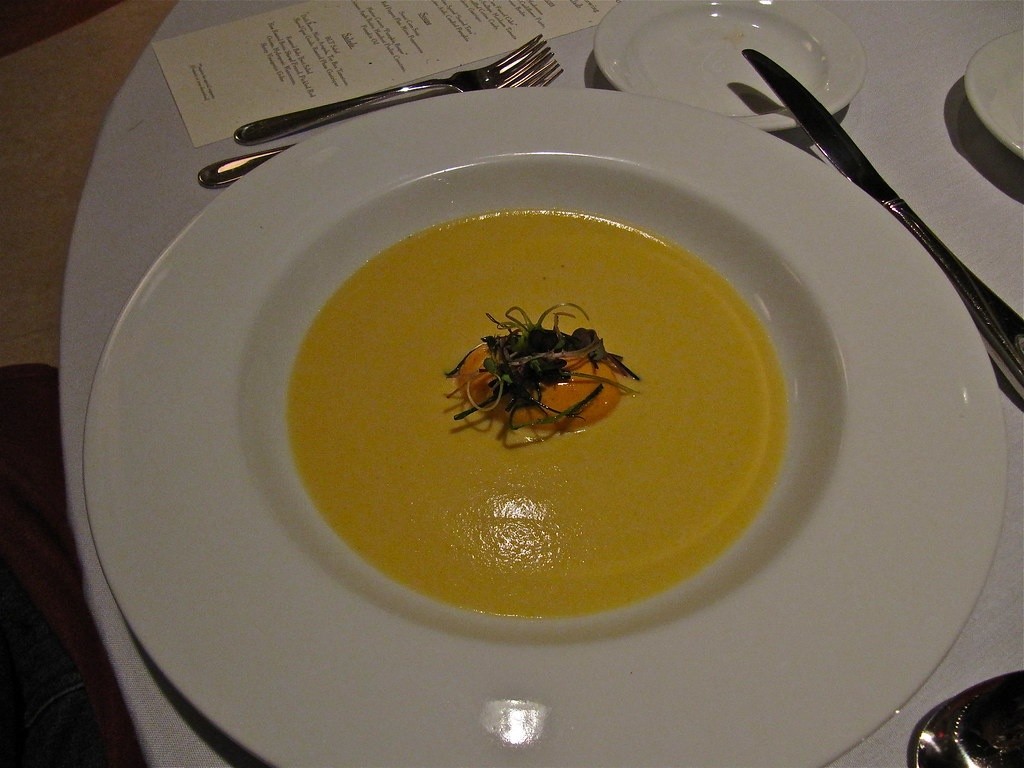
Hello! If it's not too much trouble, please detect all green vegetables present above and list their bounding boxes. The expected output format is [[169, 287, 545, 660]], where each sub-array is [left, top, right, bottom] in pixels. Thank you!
[[443, 301, 644, 431]]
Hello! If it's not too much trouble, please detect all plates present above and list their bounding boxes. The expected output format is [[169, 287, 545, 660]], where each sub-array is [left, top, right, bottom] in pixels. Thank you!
[[81, 85, 1006, 767], [594, 0, 867, 131], [964, 29, 1024, 159]]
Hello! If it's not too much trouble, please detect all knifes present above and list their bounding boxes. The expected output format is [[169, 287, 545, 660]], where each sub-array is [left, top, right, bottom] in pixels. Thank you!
[[742, 48, 1024, 399]]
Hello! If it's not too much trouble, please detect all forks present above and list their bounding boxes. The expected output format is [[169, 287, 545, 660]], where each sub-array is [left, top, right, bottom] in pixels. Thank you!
[[232, 33, 553, 146], [197, 47, 565, 190]]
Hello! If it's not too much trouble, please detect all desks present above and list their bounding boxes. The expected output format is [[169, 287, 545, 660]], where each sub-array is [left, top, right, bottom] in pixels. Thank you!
[[57, 1, 1024, 767]]
